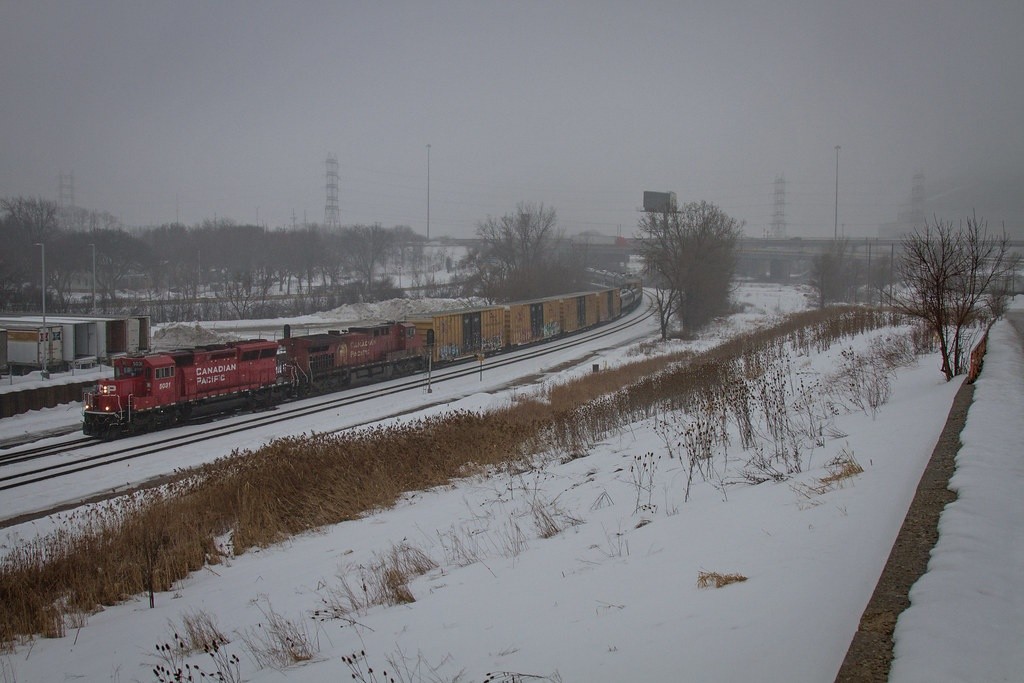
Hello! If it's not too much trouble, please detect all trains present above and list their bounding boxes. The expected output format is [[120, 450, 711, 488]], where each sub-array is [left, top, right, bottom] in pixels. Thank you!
[[80, 267, 643, 444]]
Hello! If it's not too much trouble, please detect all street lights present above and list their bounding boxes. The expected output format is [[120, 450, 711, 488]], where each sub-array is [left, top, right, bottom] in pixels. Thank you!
[[35, 242, 47, 382], [426, 143, 432, 243], [88, 242, 98, 316], [832, 143, 843, 248]]
